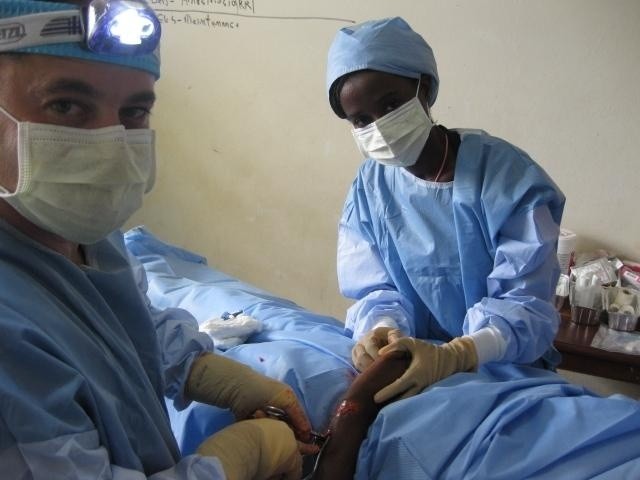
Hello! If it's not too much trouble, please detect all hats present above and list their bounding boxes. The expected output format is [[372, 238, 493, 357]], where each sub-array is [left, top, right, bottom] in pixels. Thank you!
[[329, 16, 439, 118], [1, 2, 162, 80]]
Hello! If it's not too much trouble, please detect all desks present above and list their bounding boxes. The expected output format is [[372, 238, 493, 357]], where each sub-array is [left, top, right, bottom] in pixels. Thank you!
[[553, 308, 639, 387]]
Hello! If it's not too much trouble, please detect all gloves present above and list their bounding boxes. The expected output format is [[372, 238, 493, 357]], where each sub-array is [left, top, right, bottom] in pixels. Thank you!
[[375, 335, 479, 405], [186, 352, 312, 442], [196, 418, 320, 479], [351, 327, 406, 373]]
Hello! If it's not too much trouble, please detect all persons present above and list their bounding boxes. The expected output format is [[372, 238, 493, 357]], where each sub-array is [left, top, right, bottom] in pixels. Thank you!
[[309, 345, 425, 480], [322, 14, 566, 404], [0, 0, 317, 480]]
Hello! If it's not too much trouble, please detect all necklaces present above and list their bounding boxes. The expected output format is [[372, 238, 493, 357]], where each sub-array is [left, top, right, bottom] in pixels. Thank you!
[[434, 134, 449, 182]]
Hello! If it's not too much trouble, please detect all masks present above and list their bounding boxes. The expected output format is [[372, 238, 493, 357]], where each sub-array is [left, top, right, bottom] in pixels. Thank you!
[[352, 78, 436, 168], [1, 104, 156, 245]]
